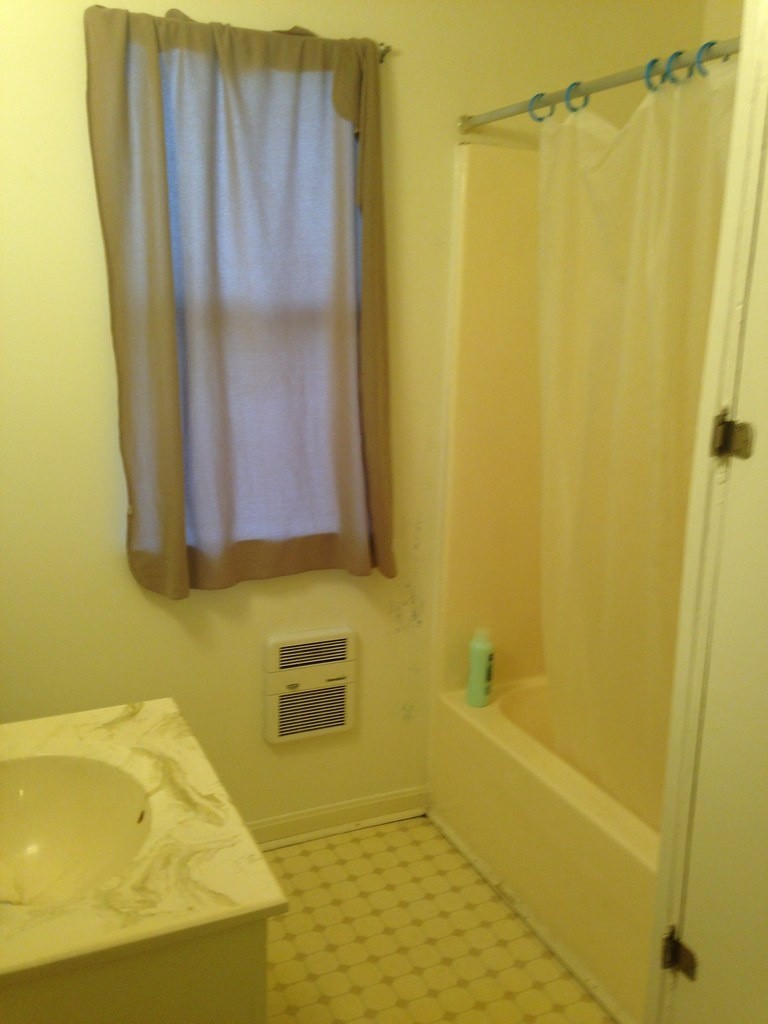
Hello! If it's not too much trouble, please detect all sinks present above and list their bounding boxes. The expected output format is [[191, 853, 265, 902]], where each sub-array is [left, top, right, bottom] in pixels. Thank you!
[[0, 752, 157, 911]]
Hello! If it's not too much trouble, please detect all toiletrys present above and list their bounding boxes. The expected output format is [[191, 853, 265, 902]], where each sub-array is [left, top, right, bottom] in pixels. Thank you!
[[465, 623, 495, 708]]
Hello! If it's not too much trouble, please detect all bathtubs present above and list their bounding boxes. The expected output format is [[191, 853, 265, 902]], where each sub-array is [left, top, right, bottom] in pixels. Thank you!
[[436, 684, 660, 1023]]
